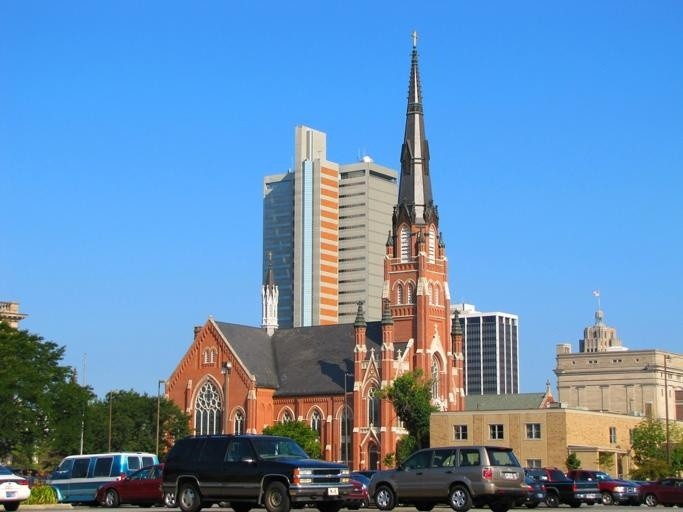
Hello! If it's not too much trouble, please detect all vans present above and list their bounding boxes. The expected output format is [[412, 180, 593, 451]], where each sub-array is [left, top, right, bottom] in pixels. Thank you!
[[44, 452, 160, 508]]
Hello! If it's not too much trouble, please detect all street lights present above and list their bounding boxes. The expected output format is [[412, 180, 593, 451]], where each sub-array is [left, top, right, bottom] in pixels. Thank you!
[[220, 361, 233, 434], [662, 354, 673, 461], [156, 380, 169, 451]]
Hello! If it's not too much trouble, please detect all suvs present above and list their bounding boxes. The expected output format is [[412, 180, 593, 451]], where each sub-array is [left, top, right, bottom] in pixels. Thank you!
[[368, 445, 641, 512], [161, 433, 355, 512]]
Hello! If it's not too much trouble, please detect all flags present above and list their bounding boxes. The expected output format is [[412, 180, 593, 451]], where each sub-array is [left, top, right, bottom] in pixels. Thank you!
[[593, 289, 600, 297]]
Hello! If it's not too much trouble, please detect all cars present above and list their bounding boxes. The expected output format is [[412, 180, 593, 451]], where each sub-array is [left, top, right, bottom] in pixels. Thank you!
[[350, 478, 368, 511], [97, 463, 179, 509], [640, 478, 683, 507], [0, 464, 31, 511]]
